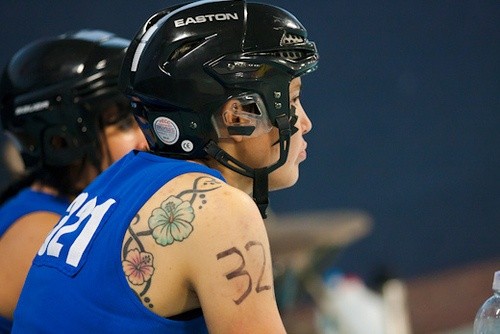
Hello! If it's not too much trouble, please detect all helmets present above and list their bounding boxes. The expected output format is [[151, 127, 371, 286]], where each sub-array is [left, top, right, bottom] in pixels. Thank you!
[[118, 0, 319, 156], [0, 30, 131, 169]]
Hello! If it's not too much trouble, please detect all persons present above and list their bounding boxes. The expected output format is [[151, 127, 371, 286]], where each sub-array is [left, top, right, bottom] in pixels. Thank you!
[[12, 0, 318, 334], [0, 30, 149, 334]]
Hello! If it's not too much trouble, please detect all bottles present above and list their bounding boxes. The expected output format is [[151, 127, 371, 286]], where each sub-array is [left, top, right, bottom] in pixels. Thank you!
[[473, 271, 500, 334]]
